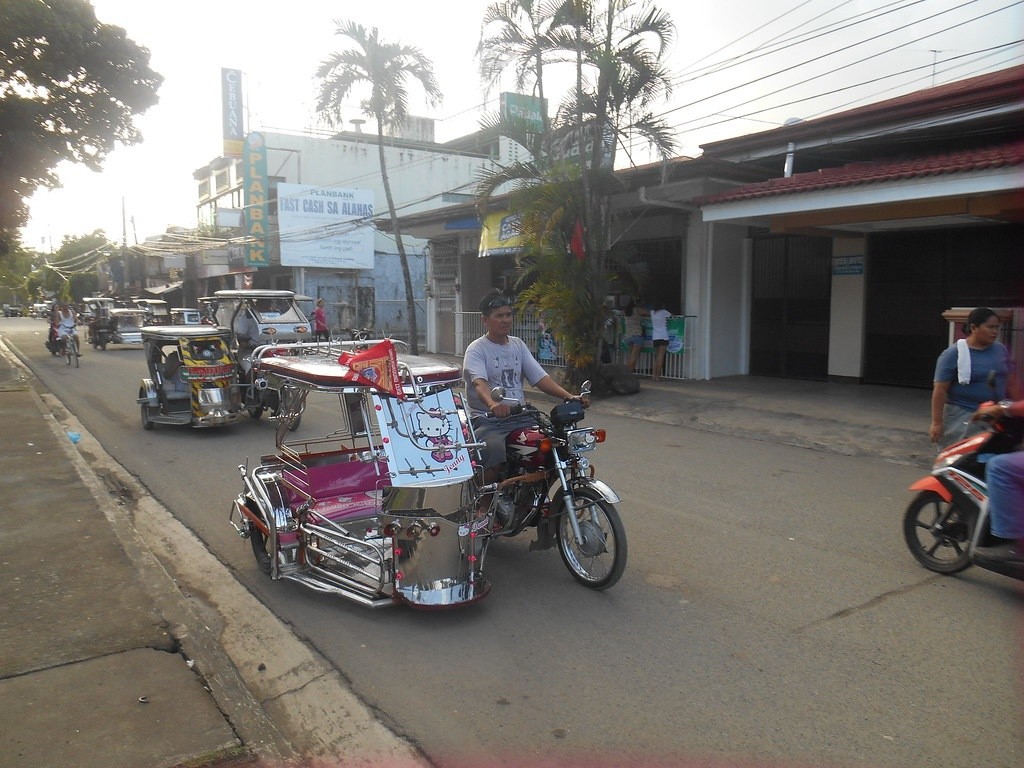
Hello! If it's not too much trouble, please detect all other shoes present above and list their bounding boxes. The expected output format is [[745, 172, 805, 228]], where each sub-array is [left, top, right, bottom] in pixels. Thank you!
[[533, 494, 552, 507], [489, 523, 503, 531], [974, 543, 1024, 562]]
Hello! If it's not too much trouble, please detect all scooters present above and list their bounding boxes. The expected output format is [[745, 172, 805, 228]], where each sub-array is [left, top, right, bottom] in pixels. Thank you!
[[2, 305, 10, 317], [342, 328, 374, 351], [44, 313, 64, 357], [904, 370, 1024, 583]]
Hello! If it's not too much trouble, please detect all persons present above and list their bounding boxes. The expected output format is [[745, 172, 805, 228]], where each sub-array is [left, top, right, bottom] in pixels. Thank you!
[[928, 308, 1010, 454], [313, 298, 328, 340], [462, 289, 592, 531], [970, 399, 1024, 547], [624, 297, 650, 370], [650, 303, 672, 382], [48, 302, 82, 357]]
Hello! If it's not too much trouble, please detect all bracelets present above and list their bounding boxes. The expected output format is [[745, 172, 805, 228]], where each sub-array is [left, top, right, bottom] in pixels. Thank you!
[[998, 400, 1014, 417]]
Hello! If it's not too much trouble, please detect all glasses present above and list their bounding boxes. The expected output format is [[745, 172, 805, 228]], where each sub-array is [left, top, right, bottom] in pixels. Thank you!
[[488, 298, 511, 309]]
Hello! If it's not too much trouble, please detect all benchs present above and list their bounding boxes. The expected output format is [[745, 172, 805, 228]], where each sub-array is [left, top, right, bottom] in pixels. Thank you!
[[159, 363, 190, 411], [282, 458, 391, 522]]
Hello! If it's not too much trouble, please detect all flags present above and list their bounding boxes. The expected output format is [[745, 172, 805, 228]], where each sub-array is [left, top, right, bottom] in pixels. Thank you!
[[337, 341, 405, 400]]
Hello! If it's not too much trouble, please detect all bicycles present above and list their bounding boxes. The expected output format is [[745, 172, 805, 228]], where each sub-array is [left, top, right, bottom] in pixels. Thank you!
[[57, 323, 80, 368]]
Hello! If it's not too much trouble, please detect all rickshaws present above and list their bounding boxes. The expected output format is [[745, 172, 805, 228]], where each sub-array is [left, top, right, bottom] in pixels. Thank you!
[[198, 296, 317, 339], [229, 336, 628, 609], [132, 299, 200, 328], [81, 297, 145, 351], [135, 288, 315, 433]]
